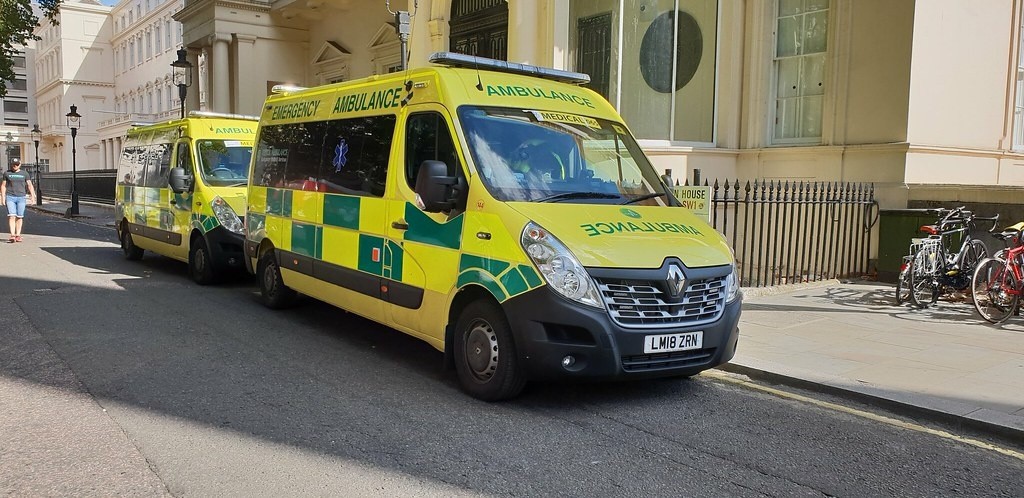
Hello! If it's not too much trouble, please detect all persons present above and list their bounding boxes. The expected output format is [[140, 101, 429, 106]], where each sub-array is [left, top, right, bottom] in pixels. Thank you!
[[0, 157, 35, 243], [509, 139, 553, 181], [119, 173, 137, 187]]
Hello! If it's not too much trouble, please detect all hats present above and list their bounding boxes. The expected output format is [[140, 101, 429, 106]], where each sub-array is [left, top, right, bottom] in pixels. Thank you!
[[10, 157, 20, 165]]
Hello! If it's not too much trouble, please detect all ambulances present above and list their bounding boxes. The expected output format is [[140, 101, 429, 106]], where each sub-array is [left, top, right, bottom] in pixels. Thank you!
[[115, 111, 260, 287], [243, 51, 743, 404]]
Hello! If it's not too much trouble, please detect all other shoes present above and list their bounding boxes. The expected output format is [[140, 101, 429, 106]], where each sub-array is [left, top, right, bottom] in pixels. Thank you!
[[15, 235, 22, 242], [9, 235, 16, 243]]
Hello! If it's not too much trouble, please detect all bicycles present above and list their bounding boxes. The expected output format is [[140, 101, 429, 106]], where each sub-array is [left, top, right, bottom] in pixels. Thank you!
[[896, 206, 1024, 323]]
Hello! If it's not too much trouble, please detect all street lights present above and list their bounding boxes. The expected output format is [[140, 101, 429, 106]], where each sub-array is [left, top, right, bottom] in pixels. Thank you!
[[5, 132, 15, 173], [169, 44, 195, 120], [65, 103, 82, 214], [31, 123, 43, 206]]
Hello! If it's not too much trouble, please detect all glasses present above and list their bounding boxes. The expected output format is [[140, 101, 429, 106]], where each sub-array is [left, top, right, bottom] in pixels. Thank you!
[[12, 163, 19, 166]]
